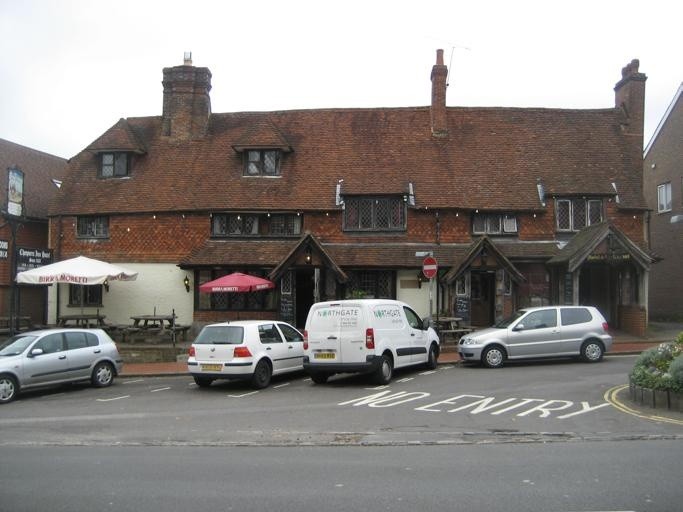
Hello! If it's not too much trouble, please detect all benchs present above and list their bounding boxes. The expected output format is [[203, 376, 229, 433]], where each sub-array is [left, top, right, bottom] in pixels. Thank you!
[[433, 323, 478, 344], [108, 320, 139, 354], [30, 321, 71, 331], [169, 323, 190, 341]]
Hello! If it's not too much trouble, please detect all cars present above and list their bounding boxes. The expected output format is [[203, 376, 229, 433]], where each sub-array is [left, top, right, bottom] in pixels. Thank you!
[[455, 305, 612, 368], [187, 320, 304, 390], [0, 328, 123, 403]]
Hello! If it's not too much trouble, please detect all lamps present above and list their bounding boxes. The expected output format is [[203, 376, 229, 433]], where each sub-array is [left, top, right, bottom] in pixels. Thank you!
[[103, 279, 110, 292], [304, 248, 312, 266], [417, 272, 422, 289], [184, 274, 189, 293]]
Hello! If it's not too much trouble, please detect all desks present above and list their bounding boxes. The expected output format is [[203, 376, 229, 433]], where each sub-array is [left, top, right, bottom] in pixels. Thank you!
[[57, 314, 107, 325], [429, 316, 463, 339], [129, 314, 178, 338]]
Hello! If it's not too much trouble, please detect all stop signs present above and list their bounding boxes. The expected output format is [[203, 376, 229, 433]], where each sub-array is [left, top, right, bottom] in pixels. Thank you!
[[422, 256, 437, 278]]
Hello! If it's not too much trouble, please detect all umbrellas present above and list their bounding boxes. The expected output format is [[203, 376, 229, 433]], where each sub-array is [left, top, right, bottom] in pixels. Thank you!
[[199, 271, 275, 320], [14, 256, 138, 328]]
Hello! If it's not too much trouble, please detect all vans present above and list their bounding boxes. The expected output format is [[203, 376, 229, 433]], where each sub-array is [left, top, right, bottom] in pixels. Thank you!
[[302, 298, 439, 385]]
